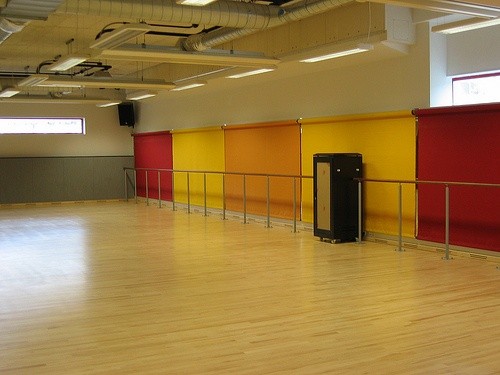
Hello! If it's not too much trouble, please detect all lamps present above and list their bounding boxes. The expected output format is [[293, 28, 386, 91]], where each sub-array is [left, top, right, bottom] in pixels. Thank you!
[[170, 80, 207, 91], [225, 66, 275, 78], [300, 47, 368, 62], [126, 91, 157, 100], [18, 74, 49, 87], [0, 88, 20, 97], [96, 101, 120, 107], [47, 38, 91, 71]]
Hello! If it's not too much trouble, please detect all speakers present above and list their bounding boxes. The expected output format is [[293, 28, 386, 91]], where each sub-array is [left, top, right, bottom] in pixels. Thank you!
[[118, 102, 135, 126]]
